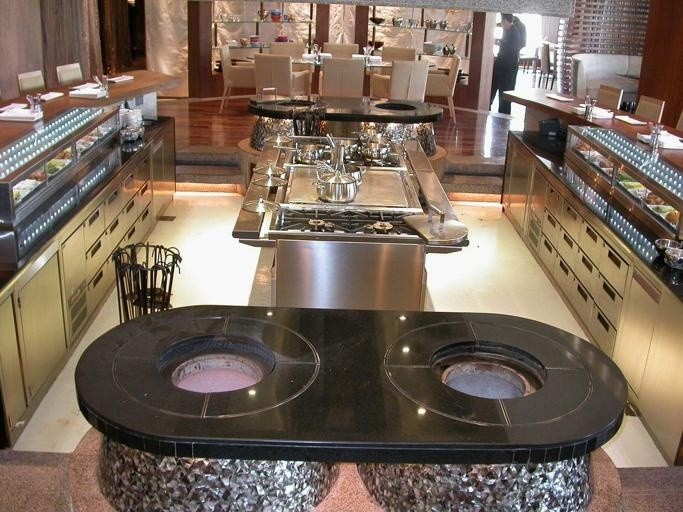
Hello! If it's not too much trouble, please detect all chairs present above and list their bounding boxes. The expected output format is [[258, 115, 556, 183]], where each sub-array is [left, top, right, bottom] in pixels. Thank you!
[[219, 42, 461, 126], [635, 96, 665, 124], [518, 42, 557, 91], [596, 84, 624, 110], [675, 110, 683, 131]]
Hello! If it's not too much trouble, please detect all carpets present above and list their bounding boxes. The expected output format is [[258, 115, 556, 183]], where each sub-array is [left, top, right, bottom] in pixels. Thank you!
[[0, 425, 682, 512]]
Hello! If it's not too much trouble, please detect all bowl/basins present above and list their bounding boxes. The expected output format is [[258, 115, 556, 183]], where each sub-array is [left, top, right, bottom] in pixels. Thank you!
[[623, 101, 629, 110], [118, 108, 143, 127], [654, 239, 682, 270], [278, 36, 288, 42], [271, 9, 281, 21], [371, 17, 385, 24], [370, 41, 383, 49], [453, 22, 473, 33]]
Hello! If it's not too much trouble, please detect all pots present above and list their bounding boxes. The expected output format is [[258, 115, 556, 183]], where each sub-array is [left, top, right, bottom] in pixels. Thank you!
[[290, 133, 392, 203]]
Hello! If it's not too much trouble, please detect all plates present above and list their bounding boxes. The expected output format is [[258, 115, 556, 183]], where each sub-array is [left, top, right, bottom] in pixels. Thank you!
[[12, 124, 112, 205], [575, 143, 679, 233]]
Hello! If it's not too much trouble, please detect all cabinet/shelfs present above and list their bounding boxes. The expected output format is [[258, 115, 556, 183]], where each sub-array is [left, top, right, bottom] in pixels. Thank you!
[[199, 0, 328, 98], [355, 6, 486, 110]]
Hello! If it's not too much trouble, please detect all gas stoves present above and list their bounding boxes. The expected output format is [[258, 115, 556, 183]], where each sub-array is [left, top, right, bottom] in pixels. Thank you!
[[266, 135, 430, 240]]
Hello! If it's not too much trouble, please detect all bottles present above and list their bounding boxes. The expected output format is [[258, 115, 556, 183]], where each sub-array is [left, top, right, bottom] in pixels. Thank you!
[[313, 45, 321, 62], [362, 47, 372, 63], [390, 17, 456, 57], [630, 96, 638, 110]]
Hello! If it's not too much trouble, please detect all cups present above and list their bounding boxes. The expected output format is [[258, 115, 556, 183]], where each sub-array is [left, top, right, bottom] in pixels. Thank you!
[[241, 38, 248, 47], [648, 121, 664, 155], [585, 94, 596, 118], [221, 14, 241, 23], [258, 10, 269, 21], [251, 36, 259, 47]]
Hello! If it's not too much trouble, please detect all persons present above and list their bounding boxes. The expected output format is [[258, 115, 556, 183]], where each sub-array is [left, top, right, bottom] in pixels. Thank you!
[[495, 14, 526, 53], [489, 12, 521, 114]]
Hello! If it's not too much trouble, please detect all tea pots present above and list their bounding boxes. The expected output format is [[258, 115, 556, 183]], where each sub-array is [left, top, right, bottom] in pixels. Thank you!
[[121, 138, 143, 154], [121, 124, 146, 140]]
